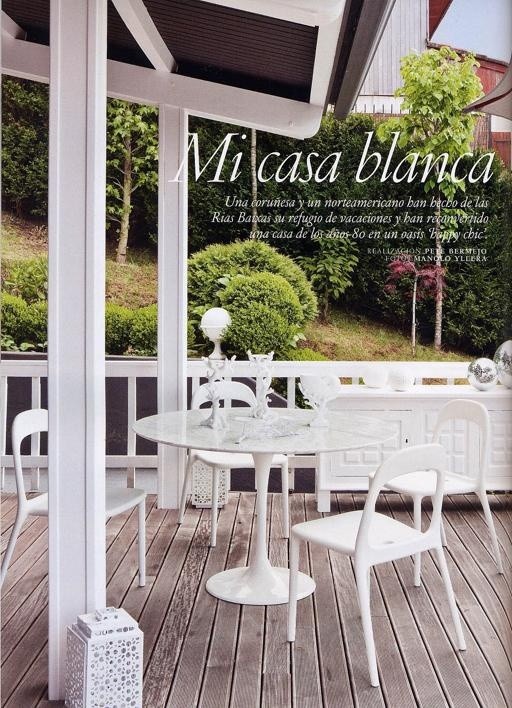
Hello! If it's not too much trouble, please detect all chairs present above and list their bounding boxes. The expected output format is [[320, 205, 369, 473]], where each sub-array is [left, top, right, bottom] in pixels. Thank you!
[[0, 404, 149, 597]]
